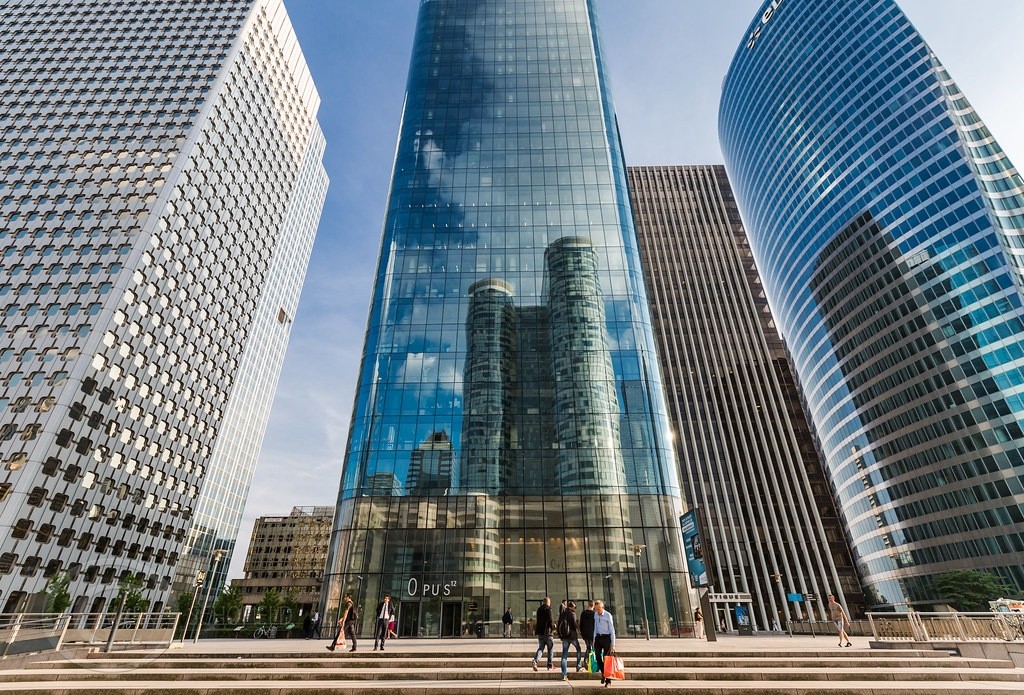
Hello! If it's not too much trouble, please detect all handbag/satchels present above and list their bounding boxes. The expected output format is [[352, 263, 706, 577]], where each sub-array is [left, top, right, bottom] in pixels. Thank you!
[[588, 646, 603, 674], [353, 611, 358, 620], [604, 650, 625, 680], [336, 627, 346, 645]]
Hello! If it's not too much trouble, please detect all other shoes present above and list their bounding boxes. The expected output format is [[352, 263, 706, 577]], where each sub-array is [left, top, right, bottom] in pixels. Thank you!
[[380, 648, 384, 650], [562, 677, 568, 681], [373, 647, 377, 650], [605, 681, 611, 687], [532, 660, 538, 671], [349, 648, 356, 652], [395, 634, 397, 637], [387, 638, 391, 640], [845, 643, 852, 647], [547, 666, 553, 670], [601, 674, 605, 684], [584, 660, 588, 671], [577, 667, 585, 672], [838, 643, 842, 647], [326, 646, 334, 651]]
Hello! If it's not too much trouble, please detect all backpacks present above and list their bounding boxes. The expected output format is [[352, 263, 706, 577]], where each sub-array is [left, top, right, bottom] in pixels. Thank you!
[[559, 611, 573, 637]]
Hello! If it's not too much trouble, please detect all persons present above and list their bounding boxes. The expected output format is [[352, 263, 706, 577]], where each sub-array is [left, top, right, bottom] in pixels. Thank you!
[[326, 593, 359, 652], [558, 602, 584, 680], [580, 601, 600, 672], [373, 594, 393, 651], [503, 607, 513, 637], [305, 610, 321, 640], [559, 599, 567, 614], [593, 600, 615, 687], [828, 595, 853, 647], [721, 619, 725, 633], [387, 614, 397, 639], [532, 597, 558, 672], [695, 608, 703, 639]]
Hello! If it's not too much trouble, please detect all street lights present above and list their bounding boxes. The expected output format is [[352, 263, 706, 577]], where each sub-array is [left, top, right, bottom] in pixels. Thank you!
[[179, 569, 206, 642], [629, 544, 651, 641], [192, 549, 230, 644], [768, 573, 793, 637]]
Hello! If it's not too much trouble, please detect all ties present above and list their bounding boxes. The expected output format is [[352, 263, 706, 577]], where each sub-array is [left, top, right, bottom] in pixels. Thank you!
[[383, 605, 386, 620]]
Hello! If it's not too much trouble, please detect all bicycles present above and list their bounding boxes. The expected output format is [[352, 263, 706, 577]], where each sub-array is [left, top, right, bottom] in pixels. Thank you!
[[253, 620, 276, 640], [988, 604, 1024, 642]]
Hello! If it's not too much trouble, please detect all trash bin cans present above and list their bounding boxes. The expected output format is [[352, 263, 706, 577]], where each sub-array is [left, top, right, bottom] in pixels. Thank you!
[[476, 623, 490, 638], [738, 625, 754, 636]]
